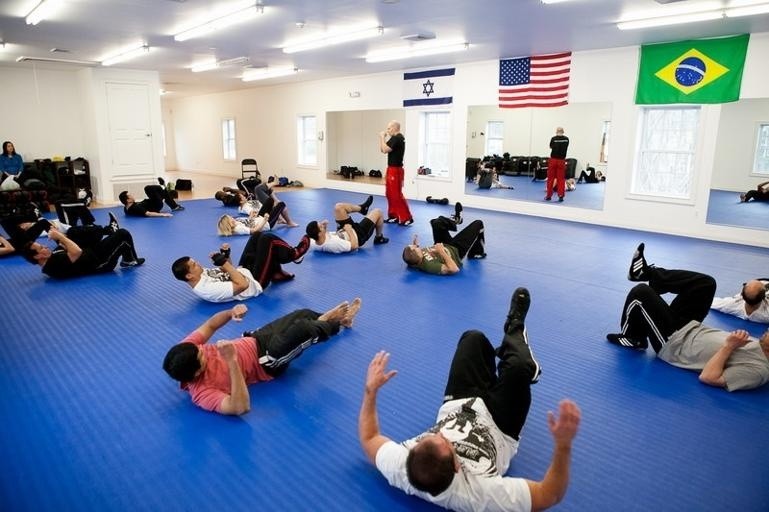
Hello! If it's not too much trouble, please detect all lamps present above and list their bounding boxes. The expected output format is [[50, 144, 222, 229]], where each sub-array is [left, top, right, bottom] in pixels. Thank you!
[[615, 0, 769, 31]]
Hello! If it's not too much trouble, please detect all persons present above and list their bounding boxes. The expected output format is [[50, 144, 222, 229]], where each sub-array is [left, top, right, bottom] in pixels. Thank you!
[[119, 177, 185, 216], [1, 141, 97, 256], [162, 298, 361, 415], [358, 287, 580, 512], [379, 120, 413, 226], [24, 211, 146, 279], [172, 231, 310, 301], [740, 182, 769, 203], [710, 277, 769, 323], [215, 170, 300, 236], [403, 202, 487, 275], [306, 195, 388, 253], [466, 127, 604, 202], [607, 243, 769, 392]]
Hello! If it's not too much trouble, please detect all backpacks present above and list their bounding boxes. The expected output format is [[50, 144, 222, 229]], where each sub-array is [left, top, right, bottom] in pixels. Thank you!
[[339, 166, 363, 179]]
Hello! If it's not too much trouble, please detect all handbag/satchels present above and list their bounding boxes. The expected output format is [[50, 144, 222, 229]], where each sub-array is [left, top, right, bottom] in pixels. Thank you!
[[268, 177, 292, 186], [368, 170, 380, 178], [173, 179, 192, 191]]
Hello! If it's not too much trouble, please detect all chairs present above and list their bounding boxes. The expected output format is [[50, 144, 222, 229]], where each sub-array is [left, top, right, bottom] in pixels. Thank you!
[[241, 158, 261, 183], [466, 155, 577, 182]]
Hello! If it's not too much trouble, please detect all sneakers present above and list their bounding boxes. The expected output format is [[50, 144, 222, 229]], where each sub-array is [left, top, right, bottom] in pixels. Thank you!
[[468, 253, 487, 259], [374, 235, 389, 245], [544, 197, 551, 200], [558, 198, 563, 201], [476, 159, 481, 168], [398, 219, 413, 227], [383, 217, 398, 223], [504, 287, 530, 333], [451, 203, 463, 224], [157, 177, 166, 191], [606, 333, 648, 352], [740, 193, 745, 203], [172, 205, 184, 211], [30, 202, 43, 220], [360, 196, 373, 215], [271, 271, 295, 282], [108, 211, 119, 235], [628, 243, 654, 281], [292, 235, 310, 265], [119, 258, 145, 269]]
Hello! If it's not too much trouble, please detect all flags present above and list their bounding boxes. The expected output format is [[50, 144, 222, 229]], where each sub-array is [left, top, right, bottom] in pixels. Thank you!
[[635, 33, 751, 105], [402, 68, 455, 107], [499, 51, 572, 109]]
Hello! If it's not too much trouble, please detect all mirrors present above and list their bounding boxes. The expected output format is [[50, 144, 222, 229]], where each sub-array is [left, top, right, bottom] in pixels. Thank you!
[[325, 108, 404, 185], [463, 104, 613, 213], [706, 98, 768, 233]]
